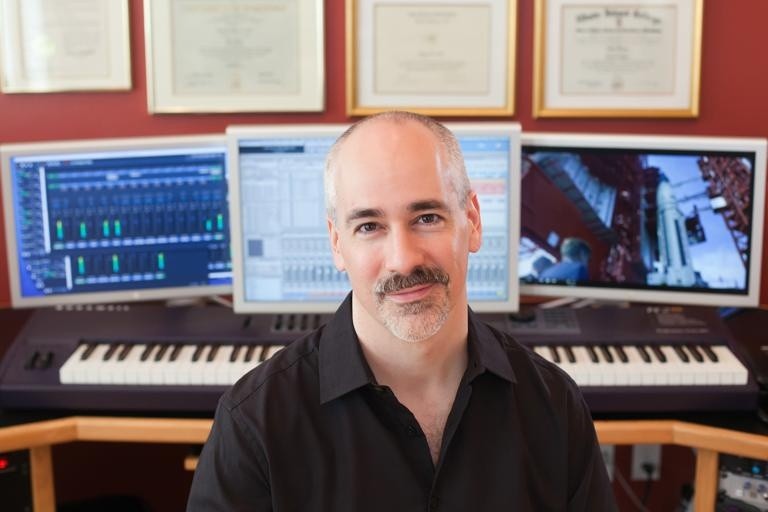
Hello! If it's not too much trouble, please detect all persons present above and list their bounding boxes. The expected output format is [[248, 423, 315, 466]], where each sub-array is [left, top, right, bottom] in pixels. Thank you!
[[184, 111, 620, 510], [539, 238, 590, 284], [692, 272, 712, 288]]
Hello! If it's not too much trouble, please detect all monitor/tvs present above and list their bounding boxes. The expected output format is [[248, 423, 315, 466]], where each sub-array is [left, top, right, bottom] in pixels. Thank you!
[[224, 122, 520, 316], [520, 131, 768, 306], [0, 133, 232, 308]]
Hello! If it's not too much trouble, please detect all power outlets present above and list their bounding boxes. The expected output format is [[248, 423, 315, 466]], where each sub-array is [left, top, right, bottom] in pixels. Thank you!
[[631, 445, 660, 482]]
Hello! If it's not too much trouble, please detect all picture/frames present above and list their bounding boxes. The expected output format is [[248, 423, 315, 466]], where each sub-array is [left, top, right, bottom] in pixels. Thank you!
[[142, 0, 325, 116], [531, 0, 705, 118], [344, 0, 518, 118], [0, 0, 134, 93]]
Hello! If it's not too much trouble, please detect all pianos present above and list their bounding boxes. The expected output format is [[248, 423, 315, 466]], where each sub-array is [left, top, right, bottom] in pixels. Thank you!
[[0, 304, 763, 411]]
